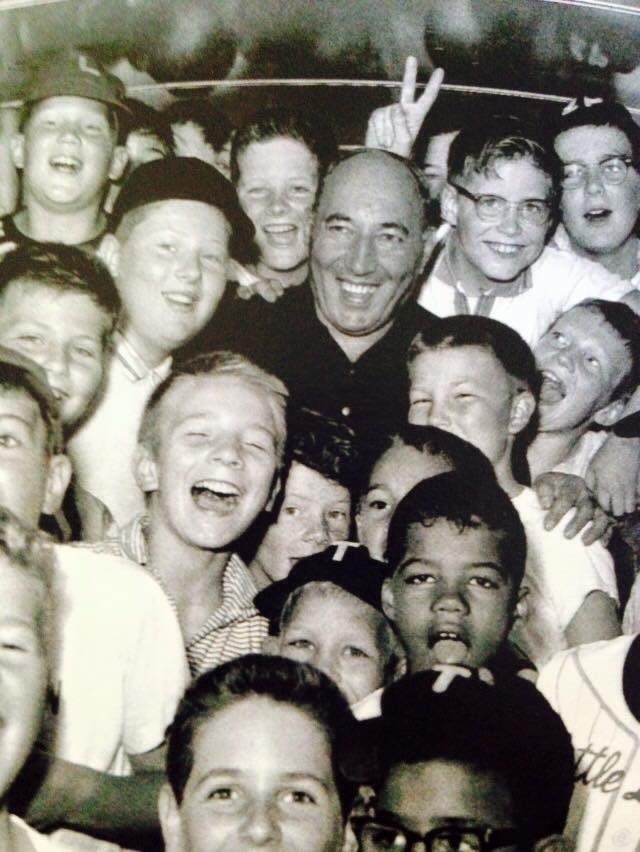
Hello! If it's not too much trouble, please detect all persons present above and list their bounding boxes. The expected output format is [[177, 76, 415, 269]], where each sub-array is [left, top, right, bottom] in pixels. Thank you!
[[0, 47, 640, 852]]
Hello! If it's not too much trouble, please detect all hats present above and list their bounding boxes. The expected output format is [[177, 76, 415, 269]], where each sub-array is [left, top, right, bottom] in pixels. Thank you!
[[359, 668, 576, 832], [110, 156, 257, 252], [253, 543, 391, 636], [25, 59, 133, 117]]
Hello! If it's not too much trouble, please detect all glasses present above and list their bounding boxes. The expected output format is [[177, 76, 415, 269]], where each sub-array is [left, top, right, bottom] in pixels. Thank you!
[[446, 180, 557, 224], [560, 159, 634, 188], [351, 816, 531, 852]]
